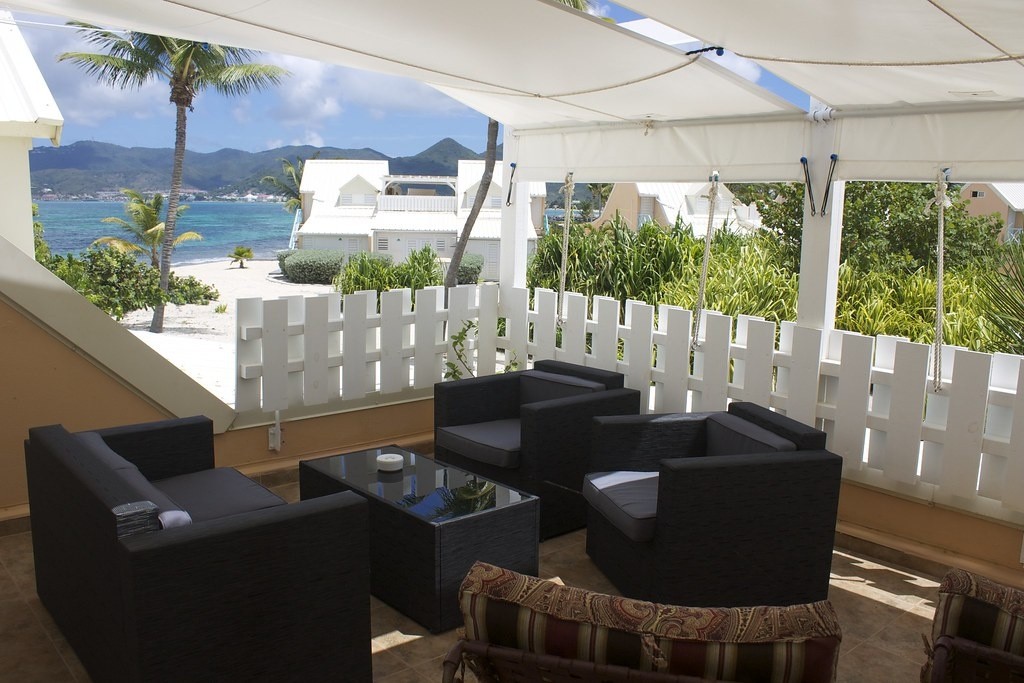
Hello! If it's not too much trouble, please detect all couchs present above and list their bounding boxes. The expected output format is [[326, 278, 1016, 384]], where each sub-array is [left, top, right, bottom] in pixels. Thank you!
[[23, 414, 373, 683], [920, 568, 1024, 683], [441, 560, 843, 683], [434, 359, 641, 543], [582, 402, 843, 609]]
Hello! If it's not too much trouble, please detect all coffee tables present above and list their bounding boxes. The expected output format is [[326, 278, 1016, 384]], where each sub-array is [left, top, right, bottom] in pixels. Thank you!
[[299, 443, 540, 635]]
